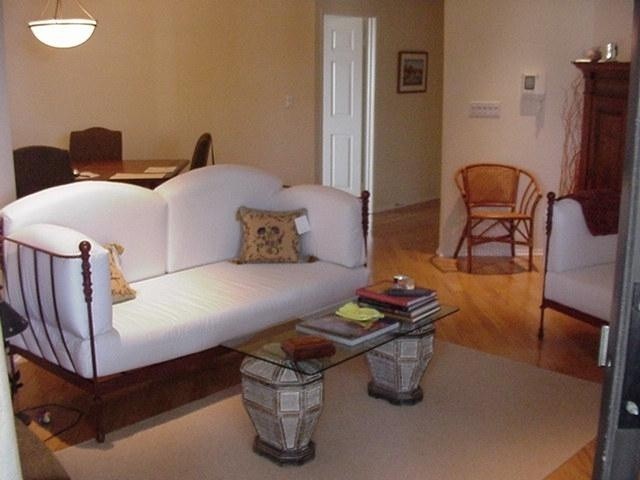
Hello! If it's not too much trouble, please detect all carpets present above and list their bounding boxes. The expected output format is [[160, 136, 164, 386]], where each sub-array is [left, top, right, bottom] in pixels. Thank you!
[[54, 339, 603, 480]]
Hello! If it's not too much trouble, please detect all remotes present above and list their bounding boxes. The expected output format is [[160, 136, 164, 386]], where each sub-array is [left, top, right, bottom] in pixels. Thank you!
[[388, 288, 431, 297]]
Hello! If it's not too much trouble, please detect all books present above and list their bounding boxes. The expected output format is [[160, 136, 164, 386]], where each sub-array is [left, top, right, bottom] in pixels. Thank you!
[[295, 308, 402, 347], [355, 279, 441, 325]]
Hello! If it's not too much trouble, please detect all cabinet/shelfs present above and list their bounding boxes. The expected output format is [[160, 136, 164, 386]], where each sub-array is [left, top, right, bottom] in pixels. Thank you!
[[571, 60, 627, 234]]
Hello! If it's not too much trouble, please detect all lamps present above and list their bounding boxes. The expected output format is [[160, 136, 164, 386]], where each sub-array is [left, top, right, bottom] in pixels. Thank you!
[[26, 0, 97, 49], [0, 299, 28, 398]]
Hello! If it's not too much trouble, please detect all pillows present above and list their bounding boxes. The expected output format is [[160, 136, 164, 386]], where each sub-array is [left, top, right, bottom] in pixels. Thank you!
[[229, 206, 318, 263], [103, 243, 136, 305]]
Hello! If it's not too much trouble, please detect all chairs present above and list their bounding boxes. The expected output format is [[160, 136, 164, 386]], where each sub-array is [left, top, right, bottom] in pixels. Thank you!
[[537, 192, 618, 340], [454, 162, 543, 273], [13, 127, 214, 198]]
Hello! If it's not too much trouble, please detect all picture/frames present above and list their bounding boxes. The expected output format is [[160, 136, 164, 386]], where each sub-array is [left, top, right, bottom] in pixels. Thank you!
[[397, 51, 428, 93]]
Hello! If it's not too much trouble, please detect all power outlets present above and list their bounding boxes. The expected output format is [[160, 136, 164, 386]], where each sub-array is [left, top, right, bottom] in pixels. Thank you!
[[470, 101, 500, 117]]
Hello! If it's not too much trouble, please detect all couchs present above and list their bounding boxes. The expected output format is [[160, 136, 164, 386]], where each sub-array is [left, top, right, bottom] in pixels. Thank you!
[[0, 185, 370, 443]]
